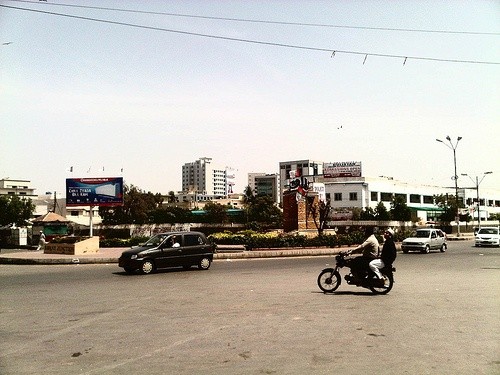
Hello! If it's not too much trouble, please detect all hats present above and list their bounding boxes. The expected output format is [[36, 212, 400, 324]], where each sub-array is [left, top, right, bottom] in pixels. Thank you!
[[40, 231, 42, 233], [388, 229, 394, 236]]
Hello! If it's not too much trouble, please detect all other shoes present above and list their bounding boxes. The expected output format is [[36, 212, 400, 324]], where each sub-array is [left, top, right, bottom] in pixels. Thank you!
[[375, 277, 385, 283]]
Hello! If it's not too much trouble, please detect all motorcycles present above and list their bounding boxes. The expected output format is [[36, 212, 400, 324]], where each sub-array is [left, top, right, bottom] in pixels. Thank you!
[[317, 251, 394, 294]]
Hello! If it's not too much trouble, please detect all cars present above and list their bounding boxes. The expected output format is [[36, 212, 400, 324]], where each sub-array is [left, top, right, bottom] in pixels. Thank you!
[[401, 228, 448, 253], [475, 227, 500, 247], [118, 231, 213, 274]]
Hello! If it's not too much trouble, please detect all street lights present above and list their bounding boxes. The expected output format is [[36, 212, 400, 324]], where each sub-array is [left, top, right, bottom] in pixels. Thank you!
[[461, 171, 493, 230]]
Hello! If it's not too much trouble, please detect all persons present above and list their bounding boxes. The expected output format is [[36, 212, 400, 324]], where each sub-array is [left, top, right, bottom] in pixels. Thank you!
[[369, 228, 397, 280], [171, 236, 180, 248], [37, 230, 46, 250], [347, 228, 379, 277]]
